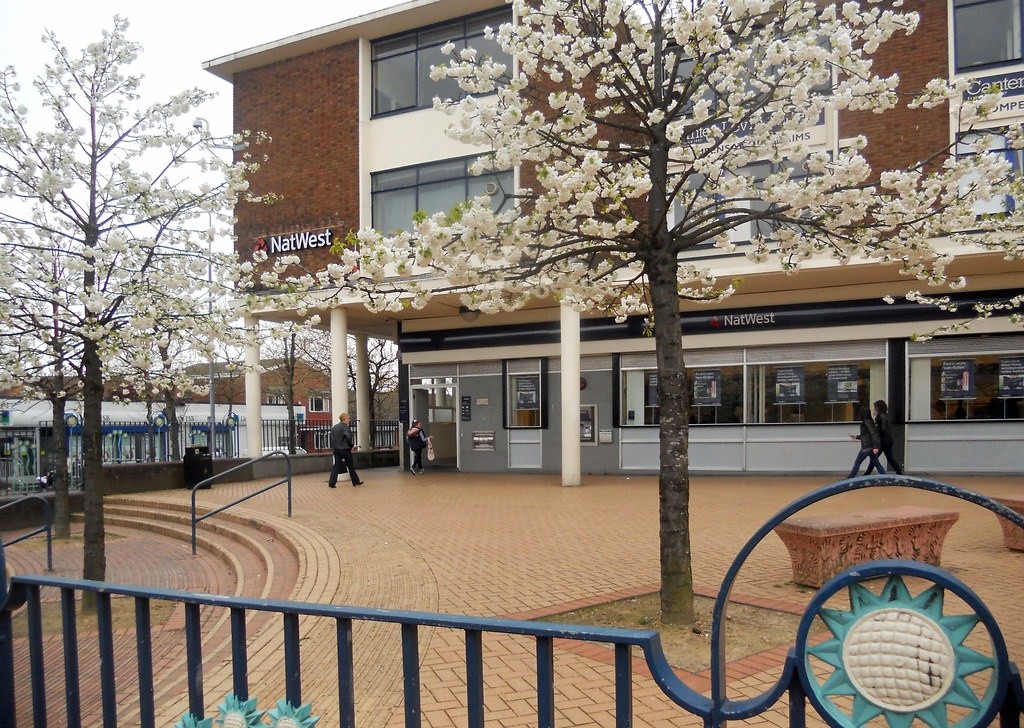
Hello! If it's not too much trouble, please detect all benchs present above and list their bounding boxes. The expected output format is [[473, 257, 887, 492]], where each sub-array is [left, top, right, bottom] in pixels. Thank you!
[[988, 496, 1024, 551], [771, 504, 959, 588]]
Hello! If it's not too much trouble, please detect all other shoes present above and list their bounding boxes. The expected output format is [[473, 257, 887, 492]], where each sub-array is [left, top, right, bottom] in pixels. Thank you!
[[354, 481, 364, 486], [410, 467, 416, 475], [418, 468, 424, 473], [329, 483, 336, 488]]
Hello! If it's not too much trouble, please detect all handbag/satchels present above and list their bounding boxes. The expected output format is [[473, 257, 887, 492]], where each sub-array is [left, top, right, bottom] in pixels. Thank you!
[[427, 437, 434, 460]]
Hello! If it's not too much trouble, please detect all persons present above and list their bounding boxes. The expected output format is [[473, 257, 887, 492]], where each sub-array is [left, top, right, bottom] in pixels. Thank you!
[[406, 420, 433, 475], [329, 413, 364, 488], [840, 406, 886, 480], [863, 399, 903, 475]]
